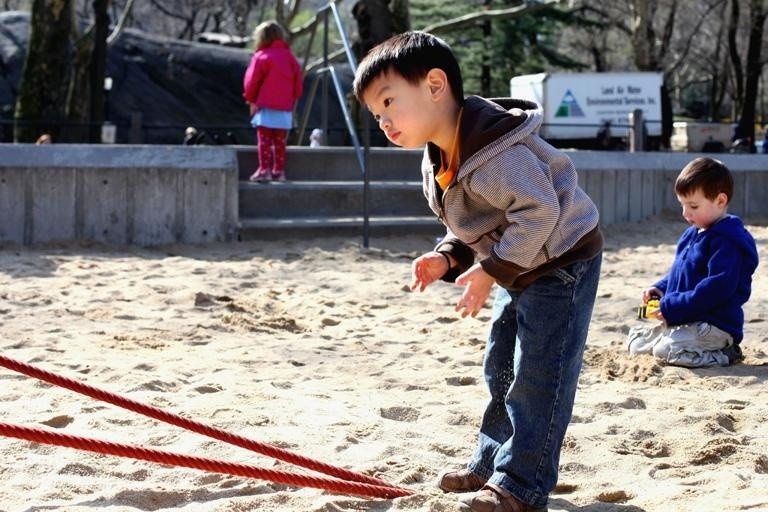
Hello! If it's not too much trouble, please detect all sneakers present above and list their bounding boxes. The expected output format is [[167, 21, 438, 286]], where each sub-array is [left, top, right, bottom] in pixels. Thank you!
[[437, 470, 488, 493], [723, 344, 743, 366], [469, 481, 549, 512], [248, 168, 273, 183], [271, 169, 286, 182]]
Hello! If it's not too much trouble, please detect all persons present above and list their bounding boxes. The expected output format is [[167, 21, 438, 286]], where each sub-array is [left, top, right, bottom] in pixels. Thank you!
[[310, 128, 324, 148], [350, 29, 605, 512], [597, 122, 611, 147], [241, 21, 303, 182], [36, 134, 50, 144], [730, 134, 753, 155], [626, 155, 759, 369], [183, 127, 196, 144]]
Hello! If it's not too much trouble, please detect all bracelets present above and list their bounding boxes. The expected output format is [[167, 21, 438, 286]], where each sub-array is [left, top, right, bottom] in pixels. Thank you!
[[439, 251, 451, 270]]
